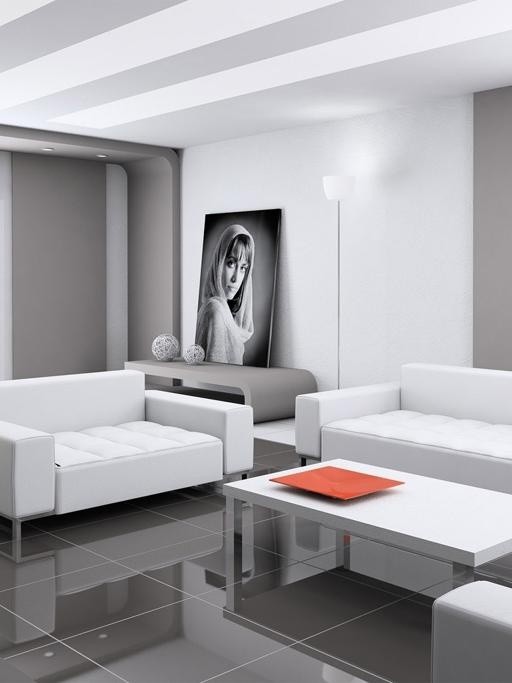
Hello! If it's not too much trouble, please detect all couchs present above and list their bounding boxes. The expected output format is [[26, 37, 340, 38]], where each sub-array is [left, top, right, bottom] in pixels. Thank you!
[[0, 361, 255, 541], [291, 361, 512, 501]]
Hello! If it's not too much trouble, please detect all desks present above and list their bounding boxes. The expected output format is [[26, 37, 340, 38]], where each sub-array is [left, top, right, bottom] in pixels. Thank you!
[[123, 358, 318, 422]]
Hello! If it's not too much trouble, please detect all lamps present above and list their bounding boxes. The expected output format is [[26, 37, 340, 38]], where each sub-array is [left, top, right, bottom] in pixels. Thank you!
[[322, 168, 352, 389]]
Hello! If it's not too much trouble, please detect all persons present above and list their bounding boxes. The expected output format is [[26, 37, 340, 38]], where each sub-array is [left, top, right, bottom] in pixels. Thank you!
[[195, 224, 256, 364]]
